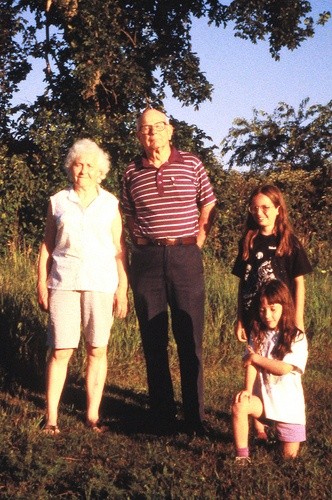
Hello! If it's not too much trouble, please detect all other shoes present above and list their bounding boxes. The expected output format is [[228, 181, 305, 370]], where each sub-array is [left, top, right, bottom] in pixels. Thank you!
[[234, 457, 251, 470], [258, 437, 267, 448], [189, 422, 210, 434]]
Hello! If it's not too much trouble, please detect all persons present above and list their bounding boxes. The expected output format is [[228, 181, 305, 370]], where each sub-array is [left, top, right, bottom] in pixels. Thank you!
[[37, 139, 128, 434], [120, 109, 217, 442], [231, 184, 313, 454], [232, 279, 308, 466]]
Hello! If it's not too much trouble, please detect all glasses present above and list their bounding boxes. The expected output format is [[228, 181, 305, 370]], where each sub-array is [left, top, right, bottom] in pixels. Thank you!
[[138, 123, 169, 132], [248, 205, 276, 213]]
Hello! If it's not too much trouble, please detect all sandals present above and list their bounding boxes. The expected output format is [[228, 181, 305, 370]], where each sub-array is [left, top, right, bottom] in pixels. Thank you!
[[85, 421, 105, 433], [42, 425, 61, 434]]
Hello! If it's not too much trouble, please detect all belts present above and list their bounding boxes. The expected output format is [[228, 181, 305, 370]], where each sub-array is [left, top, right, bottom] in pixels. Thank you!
[[136, 236, 197, 247]]
